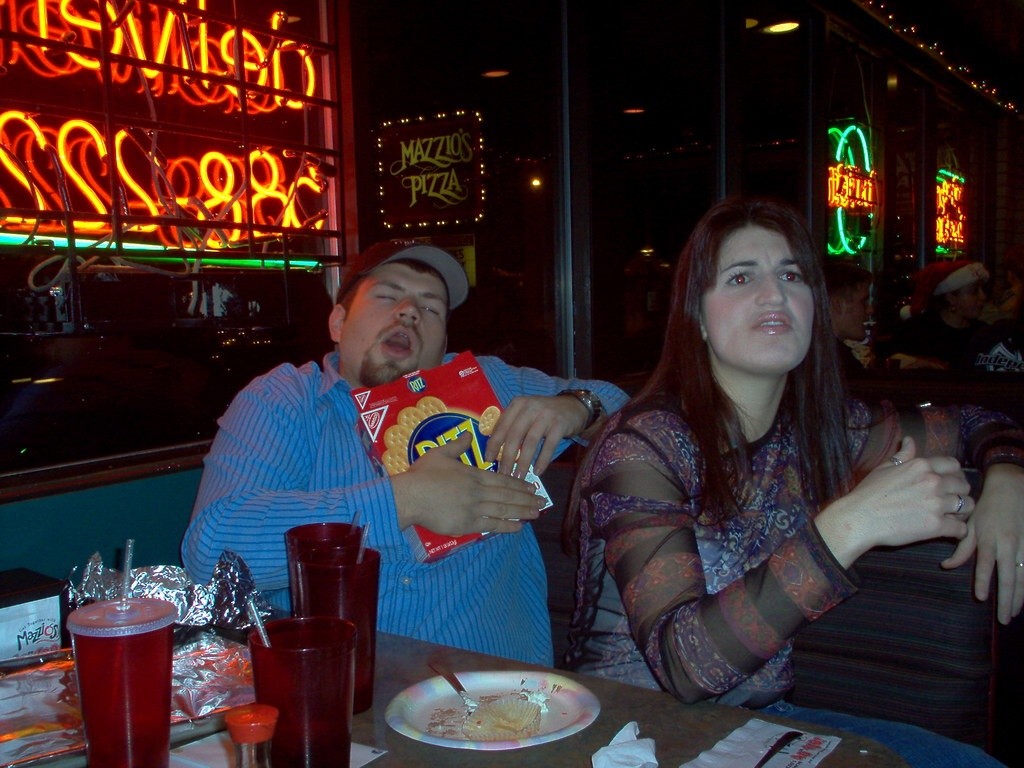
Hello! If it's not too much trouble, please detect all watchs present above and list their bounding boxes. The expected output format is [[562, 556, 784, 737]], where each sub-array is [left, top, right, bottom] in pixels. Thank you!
[[557, 389, 602, 430]]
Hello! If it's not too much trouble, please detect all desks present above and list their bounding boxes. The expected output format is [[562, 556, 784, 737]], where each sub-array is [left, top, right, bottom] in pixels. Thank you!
[[0, 565, 896, 768]]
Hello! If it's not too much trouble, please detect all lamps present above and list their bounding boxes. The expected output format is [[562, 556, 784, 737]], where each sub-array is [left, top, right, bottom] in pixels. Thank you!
[[754, 0, 800, 34]]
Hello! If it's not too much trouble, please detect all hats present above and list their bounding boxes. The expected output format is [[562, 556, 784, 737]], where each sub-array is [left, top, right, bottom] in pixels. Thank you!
[[336, 240, 468, 310], [900, 260, 989, 320]]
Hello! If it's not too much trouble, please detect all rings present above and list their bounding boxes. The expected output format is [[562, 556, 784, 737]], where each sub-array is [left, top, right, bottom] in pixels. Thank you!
[[890, 457, 903, 466], [1016, 563, 1024, 566], [957, 494, 965, 513]]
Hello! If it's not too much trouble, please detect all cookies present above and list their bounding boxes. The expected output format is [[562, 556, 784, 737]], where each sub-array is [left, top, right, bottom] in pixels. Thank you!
[[479, 405, 521, 462], [381, 396, 447, 475]]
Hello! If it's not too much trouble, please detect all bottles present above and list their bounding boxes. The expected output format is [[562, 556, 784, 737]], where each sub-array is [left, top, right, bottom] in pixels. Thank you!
[[224, 704, 280, 766]]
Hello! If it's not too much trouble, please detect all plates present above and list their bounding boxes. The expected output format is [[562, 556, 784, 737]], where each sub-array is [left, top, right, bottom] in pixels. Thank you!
[[384, 671, 602, 751]]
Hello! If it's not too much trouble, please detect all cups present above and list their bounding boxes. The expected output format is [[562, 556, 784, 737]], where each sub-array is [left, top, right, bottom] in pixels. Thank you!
[[248, 616, 359, 768], [67, 598, 178, 768], [282, 522, 364, 554], [288, 548, 381, 712]]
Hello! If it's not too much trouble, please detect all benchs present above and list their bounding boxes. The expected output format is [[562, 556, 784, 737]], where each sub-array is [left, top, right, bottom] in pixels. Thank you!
[[535, 468, 1024, 768]]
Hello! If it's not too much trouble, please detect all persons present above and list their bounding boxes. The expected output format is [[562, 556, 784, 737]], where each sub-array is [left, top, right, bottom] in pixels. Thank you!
[[562, 194, 1024, 768], [180, 239, 631, 668], [824, 241, 1024, 374]]
